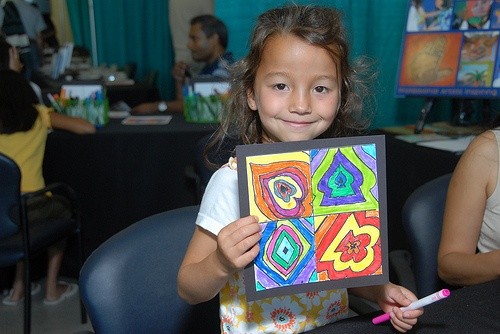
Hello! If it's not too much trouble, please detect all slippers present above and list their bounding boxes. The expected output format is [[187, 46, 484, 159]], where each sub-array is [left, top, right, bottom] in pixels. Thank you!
[[2, 283, 41, 306], [43, 280, 78, 305]]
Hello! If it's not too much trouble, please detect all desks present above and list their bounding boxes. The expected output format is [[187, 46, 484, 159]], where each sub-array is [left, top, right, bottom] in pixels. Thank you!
[[49, 112, 238, 325], [370, 120, 492, 250], [295, 280, 500, 334]]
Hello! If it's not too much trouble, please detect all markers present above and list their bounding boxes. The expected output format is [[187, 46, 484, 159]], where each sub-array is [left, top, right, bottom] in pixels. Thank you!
[[372, 289, 450, 325], [47, 93, 62, 113]]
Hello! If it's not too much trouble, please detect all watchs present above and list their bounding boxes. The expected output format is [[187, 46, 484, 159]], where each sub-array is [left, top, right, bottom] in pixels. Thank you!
[[158, 101, 168, 114]]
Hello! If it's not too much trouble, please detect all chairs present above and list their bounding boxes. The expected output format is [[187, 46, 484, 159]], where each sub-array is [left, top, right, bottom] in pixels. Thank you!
[[402, 172, 463, 301], [78, 203, 221, 334], [0, 153, 57, 334]]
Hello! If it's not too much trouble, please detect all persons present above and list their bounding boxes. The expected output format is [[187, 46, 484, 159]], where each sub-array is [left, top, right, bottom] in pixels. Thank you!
[[178, 6, 424, 334], [437, 127, 500, 288], [133, 16, 235, 207], [0, 0, 59, 82], [0, 35, 96, 305]]
